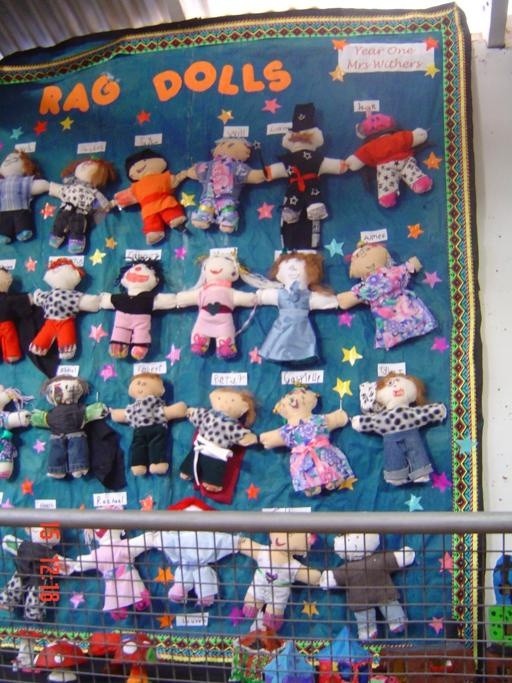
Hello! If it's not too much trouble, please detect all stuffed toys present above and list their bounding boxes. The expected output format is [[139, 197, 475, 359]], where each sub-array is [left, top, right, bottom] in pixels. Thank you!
[[1, 381, 34, 483], [186, 134, 267, 234], [344, 112, 437, 208], [350, 370, 453, 488], [254, 248, 340, 364], [262, 100, 349, 248], [257, 382, 360, 497], [47, 154, 119, 254], [0, 266, 32, 364], [72, 499, 157, 622], [234, 530, 323, 630], [110, 147, 189, 245], [176, 385, 261, 491], [29, 373, 112, 479], [155, 496, 237, 603], [25, 255, 103, 359], [1, 526, 76, 622], [0, 149, 51, 246], [109, 370, 189, 478], [175, 245, 257, 358], [336, 240, 441, 351], [96, 253, 180, 360], [318, 532, 416, 641]]
